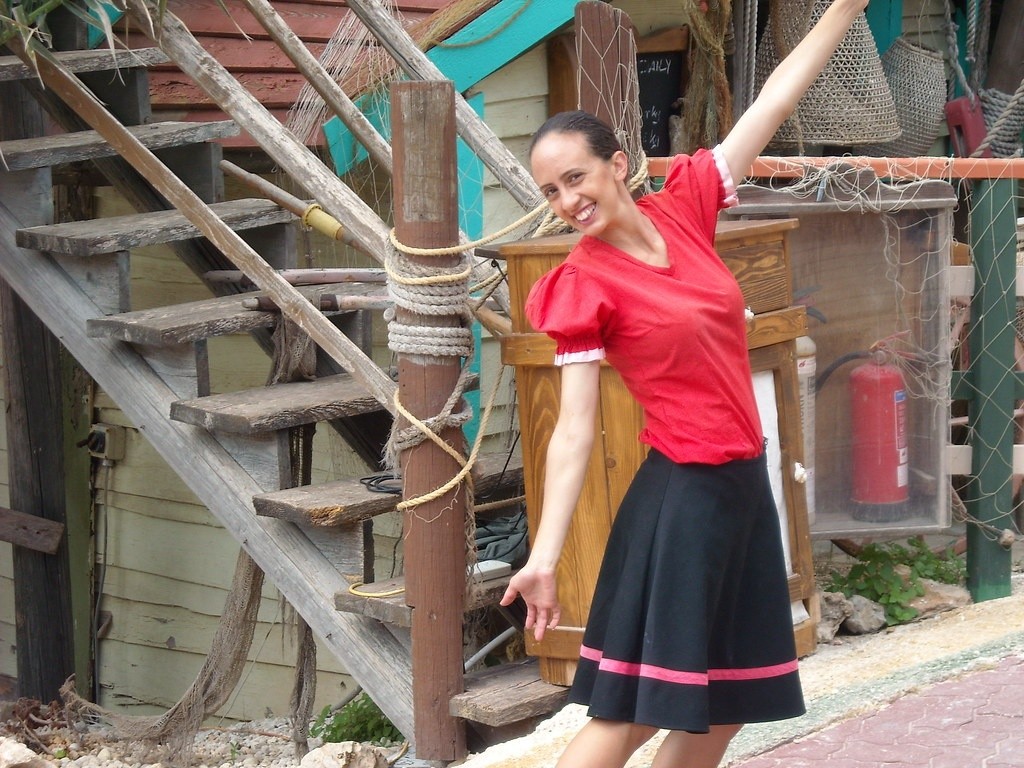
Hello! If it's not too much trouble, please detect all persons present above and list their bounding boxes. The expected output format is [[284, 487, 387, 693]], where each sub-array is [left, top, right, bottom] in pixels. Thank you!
[[499, 0, 870, 768]]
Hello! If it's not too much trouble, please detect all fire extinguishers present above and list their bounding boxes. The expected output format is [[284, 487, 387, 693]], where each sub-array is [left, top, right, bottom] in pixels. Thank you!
[[816, 330, 941, 522]]
